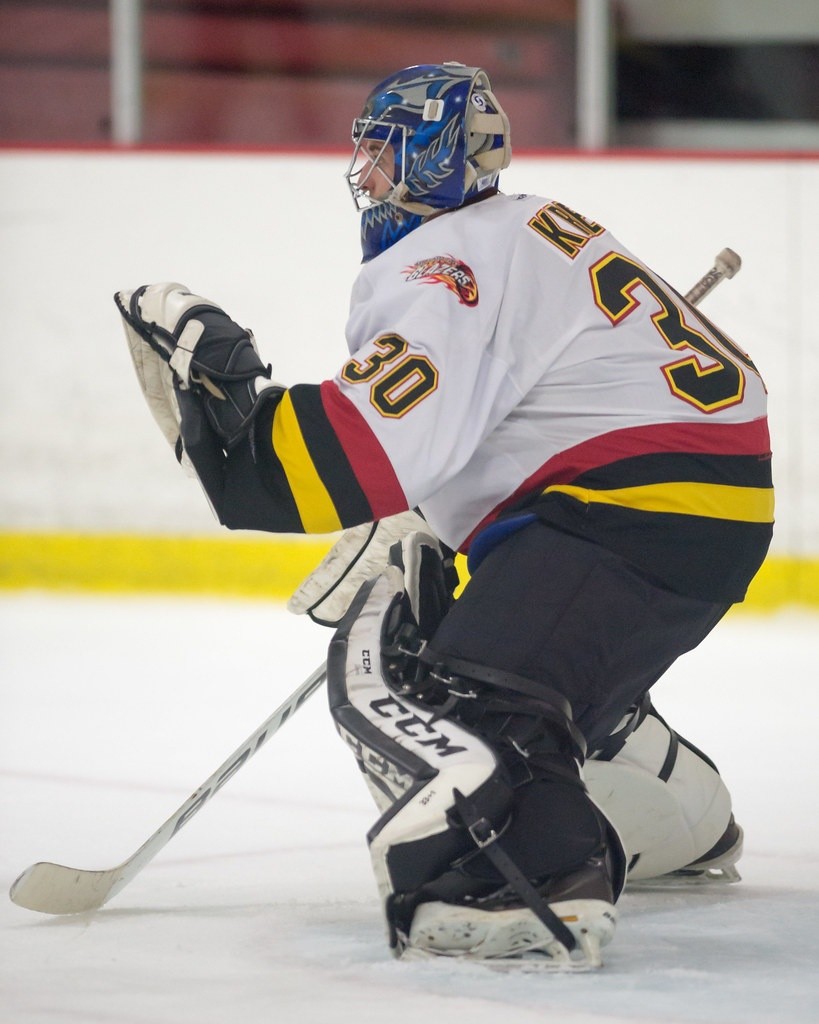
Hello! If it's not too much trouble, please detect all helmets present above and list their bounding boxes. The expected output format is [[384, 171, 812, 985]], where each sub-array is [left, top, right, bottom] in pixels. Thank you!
[[344, 62, 512, 262]]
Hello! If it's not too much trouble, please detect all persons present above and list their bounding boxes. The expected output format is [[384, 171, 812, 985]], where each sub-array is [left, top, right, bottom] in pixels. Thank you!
[[114, 63, 776, 968]]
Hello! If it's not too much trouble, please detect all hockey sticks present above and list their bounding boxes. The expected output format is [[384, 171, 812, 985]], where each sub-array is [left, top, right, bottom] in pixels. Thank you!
[[8, 243, 743, 917]]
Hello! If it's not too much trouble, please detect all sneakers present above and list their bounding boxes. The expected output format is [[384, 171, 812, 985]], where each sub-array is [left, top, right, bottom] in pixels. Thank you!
[[385, 845, 617, 973], [631, 812, 746, 889]]
